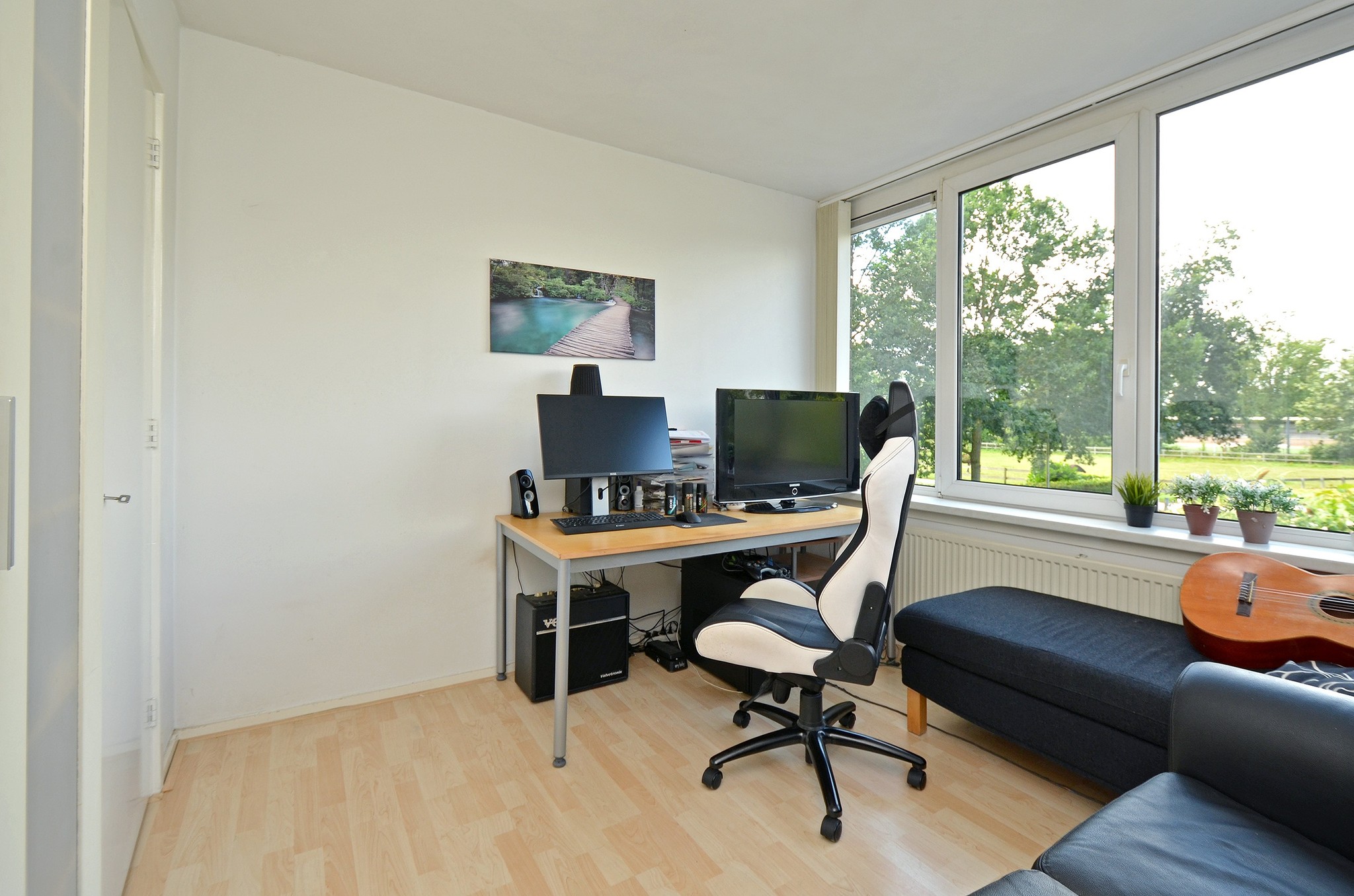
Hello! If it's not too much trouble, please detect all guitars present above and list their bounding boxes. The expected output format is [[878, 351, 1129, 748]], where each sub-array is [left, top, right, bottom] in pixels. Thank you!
[[1179, 550, 1354, 672]]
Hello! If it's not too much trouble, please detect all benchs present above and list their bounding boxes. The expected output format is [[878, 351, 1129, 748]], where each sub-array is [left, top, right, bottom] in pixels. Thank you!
[[890, 581, 1210, 785]]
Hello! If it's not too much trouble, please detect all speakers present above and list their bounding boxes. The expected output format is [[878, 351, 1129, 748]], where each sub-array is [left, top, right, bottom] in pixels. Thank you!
[[509, 469, 539, 519], [614, 475, 634, 511]]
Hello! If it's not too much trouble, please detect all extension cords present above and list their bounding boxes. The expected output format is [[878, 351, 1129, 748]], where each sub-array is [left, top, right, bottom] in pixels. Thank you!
[[639, 629, 680, 646]]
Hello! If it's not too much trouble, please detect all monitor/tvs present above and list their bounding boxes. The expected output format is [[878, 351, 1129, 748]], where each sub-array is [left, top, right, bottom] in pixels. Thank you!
[[537, 394, 674, 517], [715, 387, 860, 515]]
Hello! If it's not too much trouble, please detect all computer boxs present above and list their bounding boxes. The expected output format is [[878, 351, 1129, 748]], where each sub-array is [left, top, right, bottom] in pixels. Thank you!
[[680, 554, 792, 695], [514, 581, 629, 702]]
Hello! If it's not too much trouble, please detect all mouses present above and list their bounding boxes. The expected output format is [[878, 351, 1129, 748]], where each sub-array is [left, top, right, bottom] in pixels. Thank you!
[[676, 511, 702, 523]]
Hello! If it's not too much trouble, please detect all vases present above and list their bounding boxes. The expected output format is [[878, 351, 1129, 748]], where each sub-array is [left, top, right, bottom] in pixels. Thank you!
[[1233, 509, 1279, 546], [1181, 503, 1221, 538]]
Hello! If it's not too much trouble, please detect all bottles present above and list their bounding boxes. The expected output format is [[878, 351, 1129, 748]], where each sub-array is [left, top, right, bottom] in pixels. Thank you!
[[634, 481, 644, 511], [682, 482, 694, 513], [665, 483, 677, 515], [696, 483, 707, 513]]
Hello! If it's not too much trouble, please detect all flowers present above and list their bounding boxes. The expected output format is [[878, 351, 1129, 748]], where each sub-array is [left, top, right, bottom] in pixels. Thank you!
[[1227, 478, 1304, 516], [1162, 471, 1225, 515]]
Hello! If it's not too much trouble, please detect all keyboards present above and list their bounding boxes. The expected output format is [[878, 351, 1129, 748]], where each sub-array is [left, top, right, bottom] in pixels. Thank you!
[[550, 512, 674, 535]]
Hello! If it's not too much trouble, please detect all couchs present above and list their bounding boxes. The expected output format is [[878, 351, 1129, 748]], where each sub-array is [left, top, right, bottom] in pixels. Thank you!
[[965, 657, 1354, 896]]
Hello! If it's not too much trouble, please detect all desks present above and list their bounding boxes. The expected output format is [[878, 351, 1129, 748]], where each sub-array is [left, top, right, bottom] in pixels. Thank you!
[[491, 501, 885, 771]]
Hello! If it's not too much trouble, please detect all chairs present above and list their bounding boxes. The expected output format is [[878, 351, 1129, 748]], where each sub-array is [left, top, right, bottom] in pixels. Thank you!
[[691, 372, 934, 845]]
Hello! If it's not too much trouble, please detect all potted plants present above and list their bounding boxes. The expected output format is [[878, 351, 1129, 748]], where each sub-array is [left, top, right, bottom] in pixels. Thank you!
[[1112, 470, 1165, 529]]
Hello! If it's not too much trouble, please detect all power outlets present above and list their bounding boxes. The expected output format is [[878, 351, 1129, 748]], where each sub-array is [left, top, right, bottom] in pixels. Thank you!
[[639, 629, 681, 644]]
